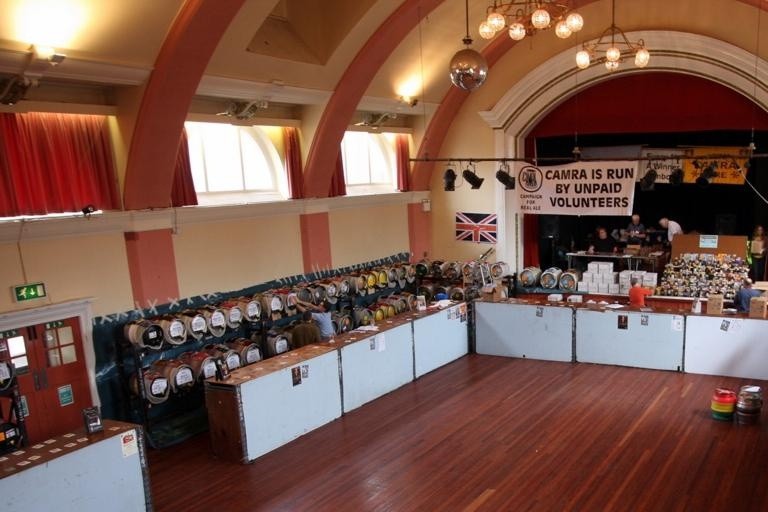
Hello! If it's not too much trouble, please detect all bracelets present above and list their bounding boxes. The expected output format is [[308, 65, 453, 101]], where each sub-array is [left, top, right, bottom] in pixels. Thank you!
[[294, 303, 298, 306]]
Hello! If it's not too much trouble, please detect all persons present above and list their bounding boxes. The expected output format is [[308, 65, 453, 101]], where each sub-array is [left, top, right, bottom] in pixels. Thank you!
[[733, 277, 762, 314], [627, 278, 655, 307], [289, 311, 324, 348], [748, 225, 768, 280], [586, 212, 683, 258], [289, 295, 335, 341]]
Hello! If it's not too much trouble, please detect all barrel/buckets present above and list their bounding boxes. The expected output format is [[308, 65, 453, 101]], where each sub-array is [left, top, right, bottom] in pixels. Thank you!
[[123, 255, 581, 404], [736, 385, 764, 425]]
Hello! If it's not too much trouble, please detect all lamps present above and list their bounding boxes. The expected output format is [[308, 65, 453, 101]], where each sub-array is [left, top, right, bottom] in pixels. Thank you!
[[439, 157, 521, 194], [576, 0, 649, 73], [637, 156, 721, 192], [478, 0, 584, 41]]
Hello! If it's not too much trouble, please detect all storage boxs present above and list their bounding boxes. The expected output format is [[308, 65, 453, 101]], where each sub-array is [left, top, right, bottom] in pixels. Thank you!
[[577, 260, 659, 295]]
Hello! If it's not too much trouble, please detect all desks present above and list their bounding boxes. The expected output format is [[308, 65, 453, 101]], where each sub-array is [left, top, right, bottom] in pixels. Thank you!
[[387, 300, 470, 379], [0, 419, 152, 511], [473, 293, 768, 381], [316, 318, 414, 414], [204, 344, 341, 464], [566, 250, 634, 271]]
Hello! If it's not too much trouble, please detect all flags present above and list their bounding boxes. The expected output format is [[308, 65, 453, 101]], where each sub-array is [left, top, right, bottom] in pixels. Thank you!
[[455, 212, 496, 244]]
